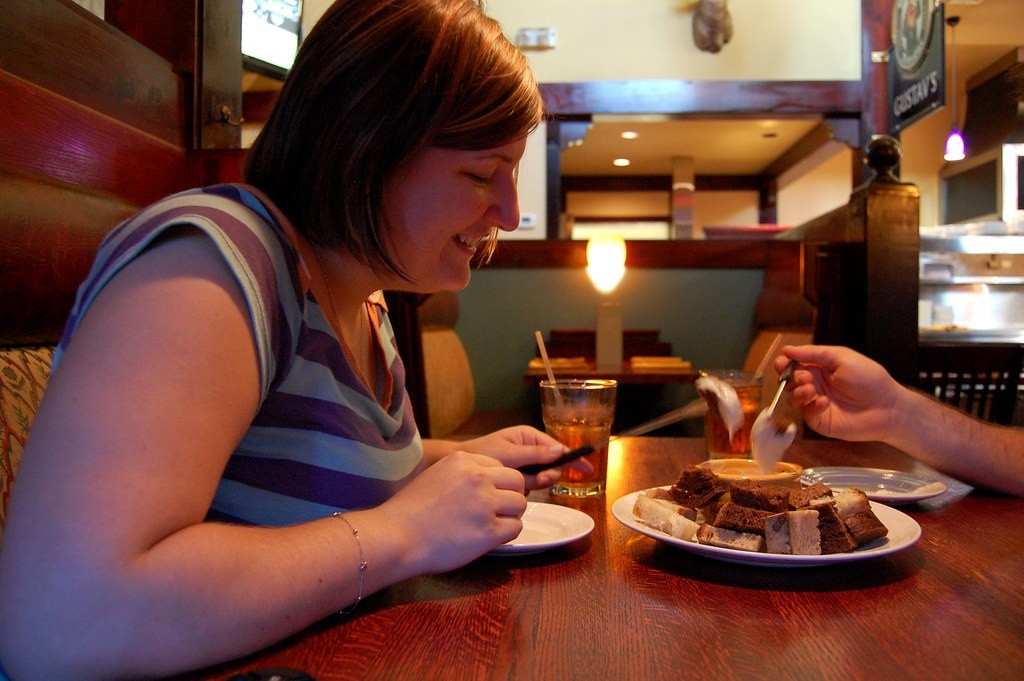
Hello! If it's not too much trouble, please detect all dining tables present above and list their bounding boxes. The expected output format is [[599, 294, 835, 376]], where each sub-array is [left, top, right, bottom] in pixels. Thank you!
[[188, 434, 1024, 681]]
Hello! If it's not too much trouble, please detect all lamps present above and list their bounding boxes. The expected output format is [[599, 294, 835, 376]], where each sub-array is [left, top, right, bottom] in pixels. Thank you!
[[945, 15, 965, 162]]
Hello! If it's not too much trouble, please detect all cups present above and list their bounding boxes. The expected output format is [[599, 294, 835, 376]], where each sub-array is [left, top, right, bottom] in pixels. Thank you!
[[698, 368, 765, 459], [541, 378, 616, 498]]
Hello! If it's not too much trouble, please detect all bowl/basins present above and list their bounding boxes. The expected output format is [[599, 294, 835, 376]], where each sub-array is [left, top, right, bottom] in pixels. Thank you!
[[696, 459, 803, 490]]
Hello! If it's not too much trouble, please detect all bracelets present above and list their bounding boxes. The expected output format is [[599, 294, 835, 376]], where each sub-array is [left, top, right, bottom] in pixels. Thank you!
[[330, 512, 367, 614]]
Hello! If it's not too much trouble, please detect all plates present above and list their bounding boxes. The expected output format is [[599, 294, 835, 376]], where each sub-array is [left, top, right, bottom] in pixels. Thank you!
[[799, 466, 946, 505], [611, 484, 922, 567], [484, 502, 594, 556]]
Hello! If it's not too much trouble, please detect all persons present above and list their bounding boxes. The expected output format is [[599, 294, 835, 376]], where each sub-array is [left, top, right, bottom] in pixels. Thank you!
[[0, 0, 595, 681], [774, 345, 1024, 497]]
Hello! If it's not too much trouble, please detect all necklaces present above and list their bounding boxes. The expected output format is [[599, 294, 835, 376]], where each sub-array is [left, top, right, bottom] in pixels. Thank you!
[[317, 251, 363, 374]]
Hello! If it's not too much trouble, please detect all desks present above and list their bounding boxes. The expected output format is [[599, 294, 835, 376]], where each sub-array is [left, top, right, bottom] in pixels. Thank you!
[[525, 355, 701, 437]]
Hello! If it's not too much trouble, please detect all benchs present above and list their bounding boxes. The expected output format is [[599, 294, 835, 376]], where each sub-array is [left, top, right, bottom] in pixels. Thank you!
[[0, 69, 188, 526], [417, 289, 540, 442], [685, 288, 817, 436]]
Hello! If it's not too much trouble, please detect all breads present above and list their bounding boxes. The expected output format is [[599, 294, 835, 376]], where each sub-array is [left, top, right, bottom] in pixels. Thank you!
[[632, 466, 888, 554]]
